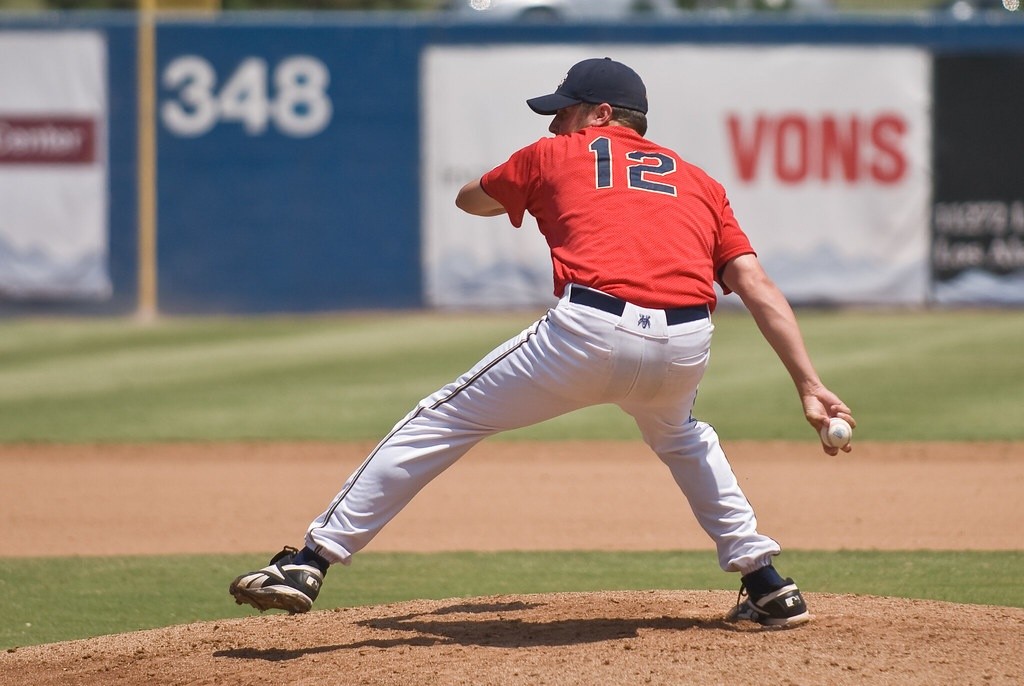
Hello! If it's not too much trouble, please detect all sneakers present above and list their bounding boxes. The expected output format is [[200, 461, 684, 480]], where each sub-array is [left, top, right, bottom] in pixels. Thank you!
[[728, 578, 809, 626], [229, 546, 324, 613]]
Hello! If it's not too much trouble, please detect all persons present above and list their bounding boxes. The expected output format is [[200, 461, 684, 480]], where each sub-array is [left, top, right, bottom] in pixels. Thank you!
[[229, 57, 857, 627]]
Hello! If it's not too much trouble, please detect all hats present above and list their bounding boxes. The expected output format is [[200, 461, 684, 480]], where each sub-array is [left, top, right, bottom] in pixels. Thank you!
[[527, 58, 648, 116]]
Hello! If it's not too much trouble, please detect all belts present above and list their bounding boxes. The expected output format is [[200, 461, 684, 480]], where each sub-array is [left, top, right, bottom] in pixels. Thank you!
[[570, 285, 709, 327]]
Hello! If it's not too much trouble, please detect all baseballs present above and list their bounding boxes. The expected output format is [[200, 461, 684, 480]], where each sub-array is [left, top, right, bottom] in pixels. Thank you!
[[820, 417, 852, 448]]
